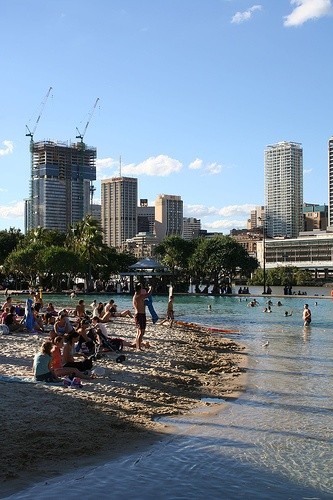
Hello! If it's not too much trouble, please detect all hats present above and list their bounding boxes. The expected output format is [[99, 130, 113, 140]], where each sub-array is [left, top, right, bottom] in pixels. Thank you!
[[80, 320, 91, 323]]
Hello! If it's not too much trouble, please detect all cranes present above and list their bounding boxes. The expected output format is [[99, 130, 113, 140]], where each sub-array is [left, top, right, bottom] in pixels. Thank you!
[[25, 87, 54, 140], [75, 97, 101, 143]]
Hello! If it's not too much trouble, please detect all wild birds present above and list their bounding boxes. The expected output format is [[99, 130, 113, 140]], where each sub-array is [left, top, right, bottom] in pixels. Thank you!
[[262, 341, 270, 347]]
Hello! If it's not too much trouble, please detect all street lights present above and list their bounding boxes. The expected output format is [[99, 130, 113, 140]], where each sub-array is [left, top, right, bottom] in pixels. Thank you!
[[258, 216, 270, 294]]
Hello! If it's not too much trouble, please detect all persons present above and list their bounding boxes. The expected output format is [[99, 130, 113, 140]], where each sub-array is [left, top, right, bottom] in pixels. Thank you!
[[132, 285, 155, 353], [0, 269, 333, 334], [33, 314, 116, 388]]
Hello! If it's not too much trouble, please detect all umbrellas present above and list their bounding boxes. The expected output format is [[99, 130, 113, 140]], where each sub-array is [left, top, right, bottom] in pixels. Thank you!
[[129, 257, 166, 269]]
